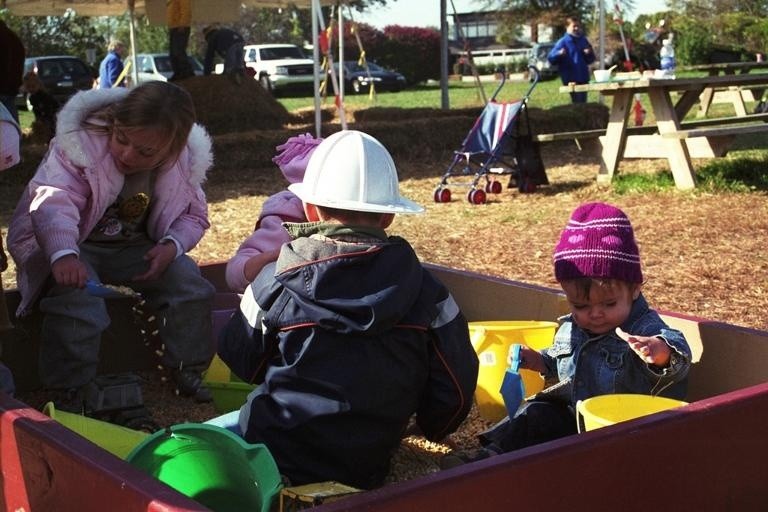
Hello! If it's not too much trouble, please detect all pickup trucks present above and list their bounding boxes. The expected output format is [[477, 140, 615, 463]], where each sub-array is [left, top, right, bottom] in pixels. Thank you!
[[215, 43, 315, 95]]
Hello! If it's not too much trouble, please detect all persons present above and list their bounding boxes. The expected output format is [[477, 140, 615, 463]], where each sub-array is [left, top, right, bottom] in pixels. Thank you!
[[548, 16, 596, 104], [478, 202, 692, 462], [202, 130, 479, 492], [165, 1, 195, 82], [1, 20, 25, 399], [202, 22, 246, 85], [7, 81, 214, 416], [99, 40, 127, 88], [20, 71, 61, 146], [225, 133, 325, 296]]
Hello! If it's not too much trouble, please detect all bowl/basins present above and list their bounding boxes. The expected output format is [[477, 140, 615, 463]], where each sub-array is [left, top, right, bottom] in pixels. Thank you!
[[594, 70, 610, 82], [204, 379, 260, 413]]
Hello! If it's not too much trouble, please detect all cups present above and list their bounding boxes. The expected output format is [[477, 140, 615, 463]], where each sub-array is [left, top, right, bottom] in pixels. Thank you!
[[756, 54, 763, 63]]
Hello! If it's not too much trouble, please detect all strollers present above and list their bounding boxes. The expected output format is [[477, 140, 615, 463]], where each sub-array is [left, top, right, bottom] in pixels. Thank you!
[[428, 61, 545, 204]]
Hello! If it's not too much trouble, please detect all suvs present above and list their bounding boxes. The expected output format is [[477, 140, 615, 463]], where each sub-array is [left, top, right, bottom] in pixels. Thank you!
[[526, 37, 563, 82]]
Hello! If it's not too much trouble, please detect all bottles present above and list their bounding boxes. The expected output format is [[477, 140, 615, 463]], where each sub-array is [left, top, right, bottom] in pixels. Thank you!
[[660, 40, 675, 79], [635, 100, 644, 126]]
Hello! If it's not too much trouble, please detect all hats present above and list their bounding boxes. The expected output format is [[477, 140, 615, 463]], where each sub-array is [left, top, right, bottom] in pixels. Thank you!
[[554, 203, 642, 282], [287, 129, 424, 214]]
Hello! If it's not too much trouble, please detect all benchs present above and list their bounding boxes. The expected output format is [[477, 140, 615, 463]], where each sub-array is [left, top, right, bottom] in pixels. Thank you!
[[522, 59, 768, 192]]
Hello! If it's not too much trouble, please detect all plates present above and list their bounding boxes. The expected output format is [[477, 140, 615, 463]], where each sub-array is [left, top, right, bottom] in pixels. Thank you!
[[612, 78, 640, 83]]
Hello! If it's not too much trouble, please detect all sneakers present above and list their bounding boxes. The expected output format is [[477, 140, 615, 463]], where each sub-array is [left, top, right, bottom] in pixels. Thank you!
[[173, 371, 212, 402], [439, 445, 504, 468]]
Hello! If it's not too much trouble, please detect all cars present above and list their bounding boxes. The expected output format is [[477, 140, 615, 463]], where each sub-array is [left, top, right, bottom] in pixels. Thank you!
[[321, 54, 405, 95], [14, 54, 96, 116]]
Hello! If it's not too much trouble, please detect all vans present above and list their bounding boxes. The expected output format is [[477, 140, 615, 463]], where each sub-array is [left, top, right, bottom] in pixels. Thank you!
[[118, 52, 205, 90]]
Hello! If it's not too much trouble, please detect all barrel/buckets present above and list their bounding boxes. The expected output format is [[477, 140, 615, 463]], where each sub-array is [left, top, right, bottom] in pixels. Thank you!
[[576, 393, 691, 435], [124, 423, 287, 512], [41, 400, 153, 461], [210, 291, 244, 357]]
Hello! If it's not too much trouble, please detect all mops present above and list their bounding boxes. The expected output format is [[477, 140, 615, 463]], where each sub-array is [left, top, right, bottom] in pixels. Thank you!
[[466, 319, 559, 422]]
